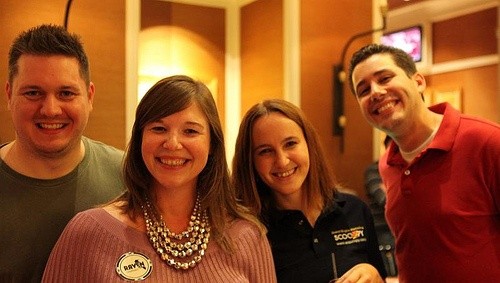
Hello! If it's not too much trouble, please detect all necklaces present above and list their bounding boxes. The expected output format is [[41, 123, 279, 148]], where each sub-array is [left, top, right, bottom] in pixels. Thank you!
[[138, 191, 212, 270]]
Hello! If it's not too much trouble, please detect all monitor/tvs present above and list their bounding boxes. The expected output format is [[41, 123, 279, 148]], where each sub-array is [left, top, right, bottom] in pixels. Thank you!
[[382, 25, 423, 63]]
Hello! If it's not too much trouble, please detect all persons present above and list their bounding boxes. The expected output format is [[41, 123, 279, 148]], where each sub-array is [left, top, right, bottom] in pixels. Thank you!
[[347, 43, 500, 283], [0, 23, 131, 283], [362, 133, 398, 277], [230, 99, 386, 283], [39, 74, 279, 283]]
